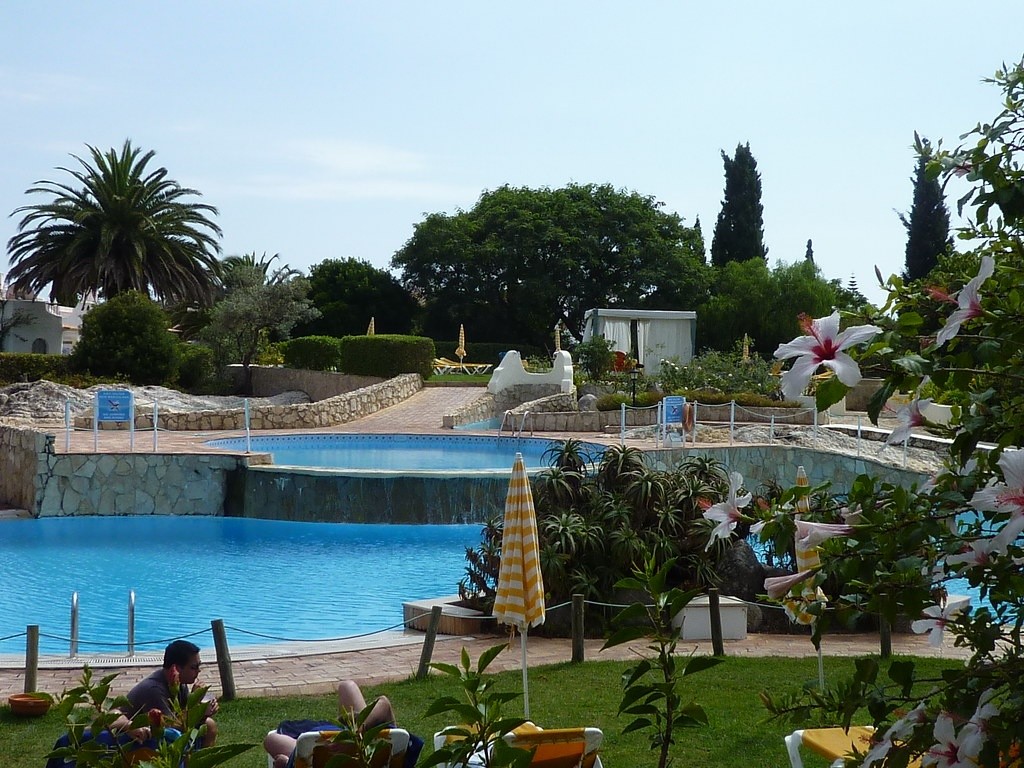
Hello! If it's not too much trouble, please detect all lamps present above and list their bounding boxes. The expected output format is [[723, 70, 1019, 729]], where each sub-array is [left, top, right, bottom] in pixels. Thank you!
[[628, 366, 640, 381]]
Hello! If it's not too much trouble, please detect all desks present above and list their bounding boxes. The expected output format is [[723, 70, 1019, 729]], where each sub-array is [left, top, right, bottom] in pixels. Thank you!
[[785, 726, 927, 768]]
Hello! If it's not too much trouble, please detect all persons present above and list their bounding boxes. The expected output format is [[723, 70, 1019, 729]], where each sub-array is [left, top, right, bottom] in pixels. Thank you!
[[91, 637, 220, 749], [263, 681, 396, 768]]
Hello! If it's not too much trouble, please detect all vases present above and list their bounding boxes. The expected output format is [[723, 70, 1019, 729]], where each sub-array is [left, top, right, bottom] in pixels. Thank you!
[[8, 694, 51, 717]]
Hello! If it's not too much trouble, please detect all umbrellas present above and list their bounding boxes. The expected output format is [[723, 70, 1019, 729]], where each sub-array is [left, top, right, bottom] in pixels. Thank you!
[[491, 451, 546, 720], [553, 325, 561, 352], [742, 333, 748, 360], [455, 324, 467, 371], [782, 466, 829, 695], [367, 317, 375, 336]]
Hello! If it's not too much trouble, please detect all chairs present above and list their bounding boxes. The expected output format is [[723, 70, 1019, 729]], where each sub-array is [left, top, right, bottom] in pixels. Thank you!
[[432, 358, 493, 375], [434, 722, 603, 768], [267, 729, 409, 768], [46, 726, 185, 768]]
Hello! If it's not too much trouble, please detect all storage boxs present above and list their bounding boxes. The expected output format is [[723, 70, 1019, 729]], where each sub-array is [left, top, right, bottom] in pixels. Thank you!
[[670, 596, 748, 641]]
